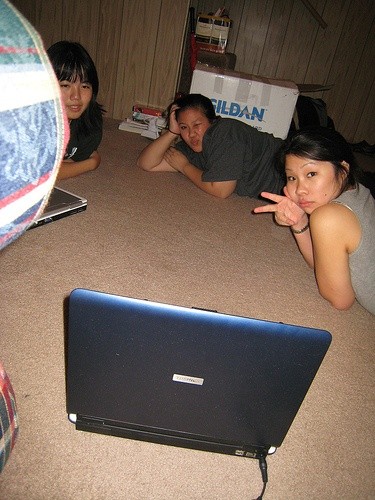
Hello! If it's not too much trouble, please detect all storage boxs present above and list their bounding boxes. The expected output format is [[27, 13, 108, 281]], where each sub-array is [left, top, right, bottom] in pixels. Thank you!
[[189, 64, 299, 140]]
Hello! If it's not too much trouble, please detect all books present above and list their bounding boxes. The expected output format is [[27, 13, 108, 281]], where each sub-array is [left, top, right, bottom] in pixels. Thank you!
[[118, 104, 168, 139]]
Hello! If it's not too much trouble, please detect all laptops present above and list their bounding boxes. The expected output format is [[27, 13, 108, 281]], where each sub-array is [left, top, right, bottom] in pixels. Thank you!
[[65, 288, 332, 459], [25, 186, 88, 231]]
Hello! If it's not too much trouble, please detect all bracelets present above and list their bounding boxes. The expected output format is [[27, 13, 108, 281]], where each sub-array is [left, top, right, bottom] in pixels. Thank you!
[[167, 128, 181, 136], [290, 223, 310, 234]]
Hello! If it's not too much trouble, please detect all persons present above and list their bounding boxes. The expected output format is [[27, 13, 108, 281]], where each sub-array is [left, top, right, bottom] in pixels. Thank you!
[[254, 126, 375, 316], [141, 96, 288, 204], [45, 41, 107, 179]]
